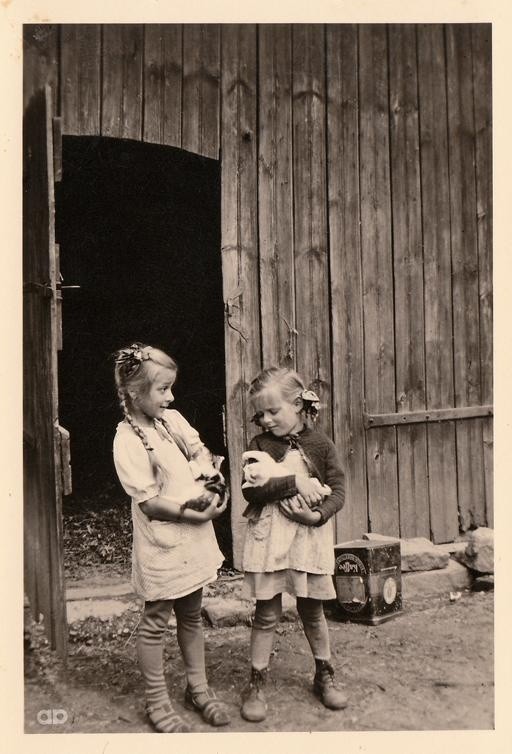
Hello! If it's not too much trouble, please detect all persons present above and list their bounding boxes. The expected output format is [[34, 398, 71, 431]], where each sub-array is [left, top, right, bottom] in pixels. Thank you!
[[241, 368, 346, 721], [113, 342, 230, 732]]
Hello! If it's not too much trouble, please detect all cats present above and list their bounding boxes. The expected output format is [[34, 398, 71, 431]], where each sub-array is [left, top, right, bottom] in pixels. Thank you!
[[179, 452, 230, 519], [241, 447, 330, 501]]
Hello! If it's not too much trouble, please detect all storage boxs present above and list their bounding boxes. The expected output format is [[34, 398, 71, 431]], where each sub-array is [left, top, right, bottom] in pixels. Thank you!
[[327, 537, 405, 627]]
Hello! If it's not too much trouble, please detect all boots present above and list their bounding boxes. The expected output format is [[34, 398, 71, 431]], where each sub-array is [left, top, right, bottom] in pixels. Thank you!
[[313, 657, 347, 709], [240, 667, 270, 722]]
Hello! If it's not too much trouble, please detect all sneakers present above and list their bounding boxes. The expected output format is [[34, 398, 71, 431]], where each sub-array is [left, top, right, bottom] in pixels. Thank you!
[[145, 700, 192, 733], [184, 684, 231, 726]]
